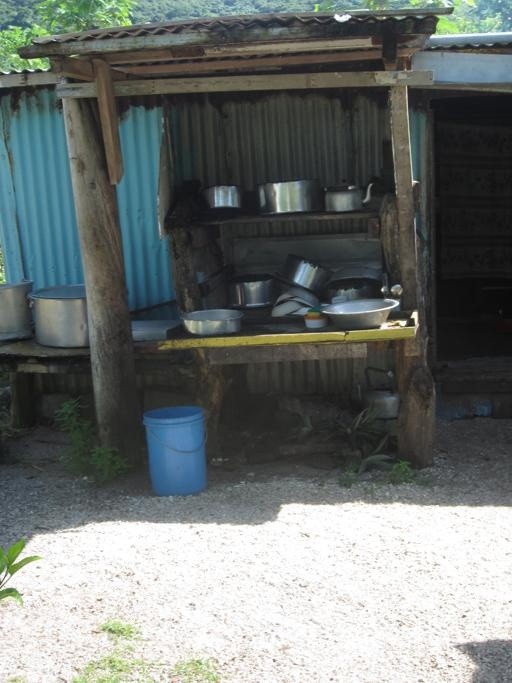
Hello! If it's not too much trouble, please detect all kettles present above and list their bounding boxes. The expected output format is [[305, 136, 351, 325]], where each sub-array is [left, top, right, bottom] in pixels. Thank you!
[[322, 179, 374, 212], [354, 366, 401, 418]]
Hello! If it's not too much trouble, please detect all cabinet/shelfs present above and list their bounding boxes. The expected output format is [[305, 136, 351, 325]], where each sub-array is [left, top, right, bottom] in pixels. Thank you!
[[157, 209, 416, 319]]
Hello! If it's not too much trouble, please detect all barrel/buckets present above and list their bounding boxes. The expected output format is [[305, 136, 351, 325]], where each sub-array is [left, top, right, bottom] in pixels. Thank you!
[[142, 406, 208, 498]]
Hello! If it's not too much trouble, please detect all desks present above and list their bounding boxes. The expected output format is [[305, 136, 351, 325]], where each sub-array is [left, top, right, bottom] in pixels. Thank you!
[[0, 322, 433, 472]]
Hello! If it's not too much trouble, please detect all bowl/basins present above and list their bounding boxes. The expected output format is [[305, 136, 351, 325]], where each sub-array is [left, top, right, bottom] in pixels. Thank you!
[[257, 179, 319, 216], [276, 292, 297, 306], [233, 274, 276, 308], [287, 297, 313, 307], [200, 185, 244, 209], [320, 298, 400, 331], [327, 266, 384, 288], [286, 253, 335, 294], [272, 301, 302, 317], [284, 286, 320, 307], [291, 307, 312, 315], [179, 308, 245, 336]]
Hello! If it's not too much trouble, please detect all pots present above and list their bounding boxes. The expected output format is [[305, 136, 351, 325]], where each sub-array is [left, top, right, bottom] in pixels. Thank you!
[[28, 285, 91, 348], [1, 280, 34, 340]]
